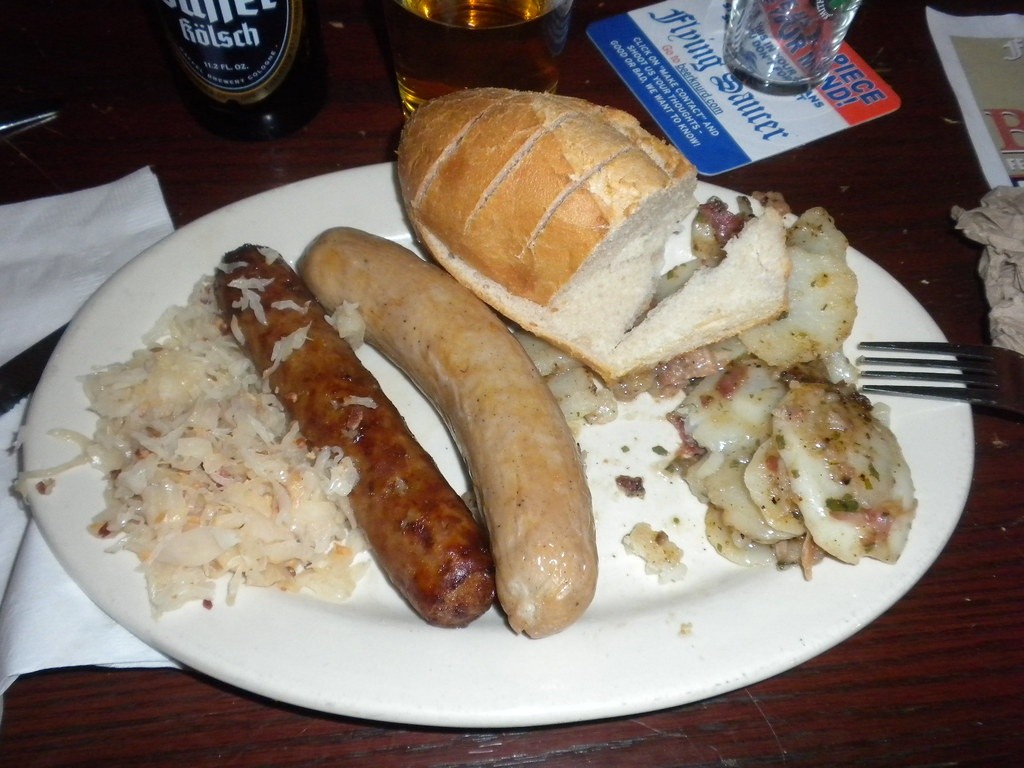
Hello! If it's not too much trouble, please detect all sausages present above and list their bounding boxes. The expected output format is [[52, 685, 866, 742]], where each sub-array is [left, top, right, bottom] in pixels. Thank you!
[[215, 244, 498, 633], [298, 225, 598, 640]]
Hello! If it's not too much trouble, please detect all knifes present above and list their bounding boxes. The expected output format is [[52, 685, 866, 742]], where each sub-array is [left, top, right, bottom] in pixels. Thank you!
[[0, 322, 70, 418]]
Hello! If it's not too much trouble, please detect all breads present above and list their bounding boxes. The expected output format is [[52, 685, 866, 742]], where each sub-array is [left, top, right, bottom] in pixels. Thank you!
[[393, 85, 789, 390]]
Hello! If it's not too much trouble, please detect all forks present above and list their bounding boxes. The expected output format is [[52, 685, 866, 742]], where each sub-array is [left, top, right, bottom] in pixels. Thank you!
[[853, 336, 1024, 423]]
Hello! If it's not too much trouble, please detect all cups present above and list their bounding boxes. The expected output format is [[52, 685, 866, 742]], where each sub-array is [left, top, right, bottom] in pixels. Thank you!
[[383, 0, 574, 146], [723, 0, 861, 98]]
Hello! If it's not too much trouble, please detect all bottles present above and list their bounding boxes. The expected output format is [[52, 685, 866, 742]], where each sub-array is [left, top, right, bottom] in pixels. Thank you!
[[154, 0, 326, 142]]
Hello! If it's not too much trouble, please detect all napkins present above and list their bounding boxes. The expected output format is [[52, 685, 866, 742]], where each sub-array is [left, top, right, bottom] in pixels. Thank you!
[[0, 164, 182, 696]]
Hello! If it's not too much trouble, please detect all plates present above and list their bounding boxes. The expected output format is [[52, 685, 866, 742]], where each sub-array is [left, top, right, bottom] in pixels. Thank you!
[[20, 160, 977, 728]]
